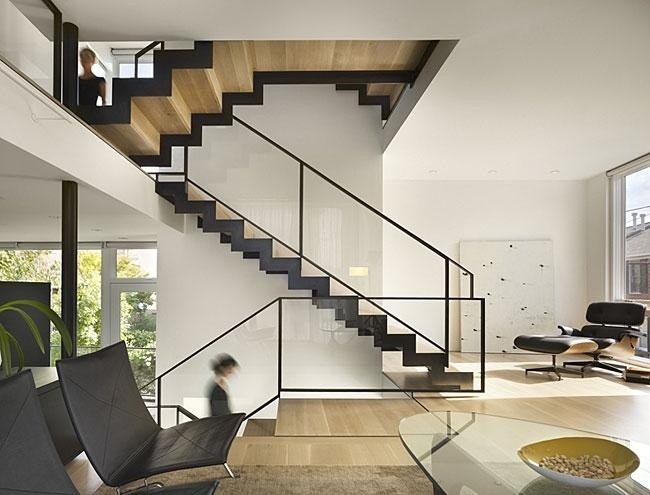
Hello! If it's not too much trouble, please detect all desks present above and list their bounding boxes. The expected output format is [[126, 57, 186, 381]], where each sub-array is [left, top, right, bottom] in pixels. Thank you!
[[396, 409, 649, 494]]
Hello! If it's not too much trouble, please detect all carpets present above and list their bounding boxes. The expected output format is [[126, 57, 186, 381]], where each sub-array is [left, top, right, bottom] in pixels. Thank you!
[[91, 464, 612, 494]]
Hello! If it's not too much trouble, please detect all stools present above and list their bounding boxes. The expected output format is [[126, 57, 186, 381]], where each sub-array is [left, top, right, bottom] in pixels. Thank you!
[[513, 334, 599, 383]]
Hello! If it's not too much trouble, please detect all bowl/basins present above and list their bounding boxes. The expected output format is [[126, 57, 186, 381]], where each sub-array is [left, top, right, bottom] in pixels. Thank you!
[[519, 435, 641, 487]]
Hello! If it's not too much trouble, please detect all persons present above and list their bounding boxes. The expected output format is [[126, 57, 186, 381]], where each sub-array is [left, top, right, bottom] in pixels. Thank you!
[[208, 354, 239, 416], [79, 48, 106, 107]]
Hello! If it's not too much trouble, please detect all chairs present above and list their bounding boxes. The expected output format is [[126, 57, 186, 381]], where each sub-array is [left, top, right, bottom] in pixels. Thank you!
[[52, 338, 248, 494], [555, 301, 649, 380], [1, 366, 221, 494]]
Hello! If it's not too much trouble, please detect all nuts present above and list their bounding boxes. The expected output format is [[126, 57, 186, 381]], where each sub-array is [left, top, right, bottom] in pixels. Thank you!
[[539, 454, 615, 479]]
